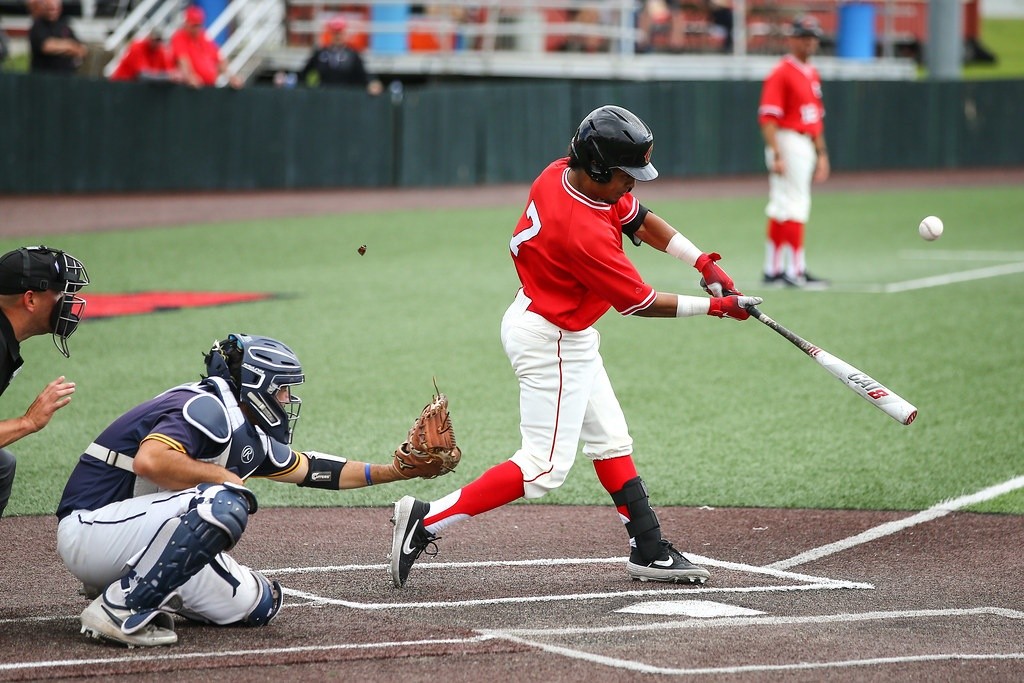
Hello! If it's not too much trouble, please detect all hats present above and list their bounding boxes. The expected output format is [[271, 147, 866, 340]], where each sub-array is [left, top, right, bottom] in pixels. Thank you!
[[186, 6, 205, 24], [0, 244, 65, 295], [791, 15, 823, 40], [328, 17, 345, 33]]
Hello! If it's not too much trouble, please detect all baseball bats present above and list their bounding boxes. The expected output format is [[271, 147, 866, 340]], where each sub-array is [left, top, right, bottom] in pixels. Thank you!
[[699, 277, 919, 427]]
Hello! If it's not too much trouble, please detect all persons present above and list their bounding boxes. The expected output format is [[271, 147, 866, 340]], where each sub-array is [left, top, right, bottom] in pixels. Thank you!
[[389, 106, 764, 590], [757, 14, 835, 291], [0, 245, 89, 519], [25, 0, 734, 95], [57, 333, 461, 649]]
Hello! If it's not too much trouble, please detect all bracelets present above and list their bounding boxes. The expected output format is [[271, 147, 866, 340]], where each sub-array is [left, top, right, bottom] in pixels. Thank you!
[[817, 147, 828, 155], [365, 463, 373, 487]]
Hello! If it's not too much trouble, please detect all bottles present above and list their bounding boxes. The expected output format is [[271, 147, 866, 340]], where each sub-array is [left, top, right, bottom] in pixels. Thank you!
[[389, 78, 402, 105], [273, 71, 298, 89]]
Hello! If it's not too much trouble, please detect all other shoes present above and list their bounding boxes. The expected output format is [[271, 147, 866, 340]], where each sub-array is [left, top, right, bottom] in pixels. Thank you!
[[765, 273, 827, 292]]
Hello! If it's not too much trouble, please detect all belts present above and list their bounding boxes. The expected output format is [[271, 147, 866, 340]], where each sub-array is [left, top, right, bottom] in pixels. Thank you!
[[527, 302, 540, 313]]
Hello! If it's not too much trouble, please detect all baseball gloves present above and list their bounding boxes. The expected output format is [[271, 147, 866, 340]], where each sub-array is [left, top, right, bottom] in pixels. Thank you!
[[389, 375, 463, 483]]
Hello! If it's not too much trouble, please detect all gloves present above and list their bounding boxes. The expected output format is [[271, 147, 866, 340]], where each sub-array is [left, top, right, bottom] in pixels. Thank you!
[[695, 253, 741, 300], [706, 290, 763, 322]]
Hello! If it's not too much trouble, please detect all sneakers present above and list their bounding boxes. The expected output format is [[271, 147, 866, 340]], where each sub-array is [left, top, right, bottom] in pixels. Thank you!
[[387, 494, 443, 588], [626, 540, 712, 584], [77, 593, 178, 649]]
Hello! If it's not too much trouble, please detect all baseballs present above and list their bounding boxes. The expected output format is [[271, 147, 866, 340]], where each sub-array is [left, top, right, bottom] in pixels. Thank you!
[[918, 215, 945, 242]]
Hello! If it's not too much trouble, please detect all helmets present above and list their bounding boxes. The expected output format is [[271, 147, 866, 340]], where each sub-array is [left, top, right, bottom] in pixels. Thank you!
[[572, 105, 659, 183], [204, 333, 305, 446]]
